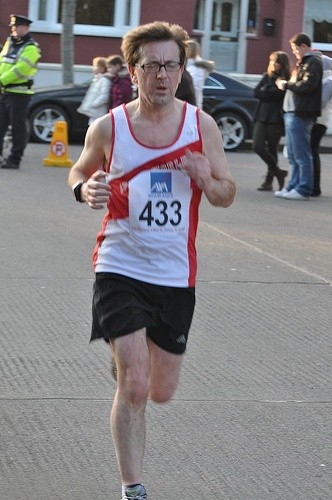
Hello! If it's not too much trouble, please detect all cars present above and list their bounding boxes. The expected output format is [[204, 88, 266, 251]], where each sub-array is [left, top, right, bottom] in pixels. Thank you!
[[27, 66, 260, 153]]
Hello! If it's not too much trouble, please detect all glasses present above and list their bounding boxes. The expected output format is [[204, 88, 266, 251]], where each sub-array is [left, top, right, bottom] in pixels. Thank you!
[[134, 61, 183, 73]]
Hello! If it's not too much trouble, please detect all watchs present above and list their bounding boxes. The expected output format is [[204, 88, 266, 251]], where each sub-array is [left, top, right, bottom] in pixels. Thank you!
[[73, 181, 84, 203]]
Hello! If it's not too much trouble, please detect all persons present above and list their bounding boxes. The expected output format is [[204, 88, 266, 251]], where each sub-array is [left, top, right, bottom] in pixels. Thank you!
[[76, 56, 112, 127], [0, 15, 42, 168], [185, 41, 215, 111], [252, 51, 290, 192], [274, 32, 323, 200], [107, 56, 133, 108], [311, 48, 332, 197], [67, 21, 236, 500]]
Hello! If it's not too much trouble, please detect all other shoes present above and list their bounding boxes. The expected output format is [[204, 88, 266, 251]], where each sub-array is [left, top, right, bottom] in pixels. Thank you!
[[0, 160, 19, 168], [274, 188, 288, 198], [121, 483, 147, 500], [283, 188, 307, 200], [258, 184, 272, 190], [276, 170, 288, 190]]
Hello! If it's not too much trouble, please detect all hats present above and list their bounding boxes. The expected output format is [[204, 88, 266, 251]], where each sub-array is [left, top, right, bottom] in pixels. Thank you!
[[8, 14, 32, 27]]
